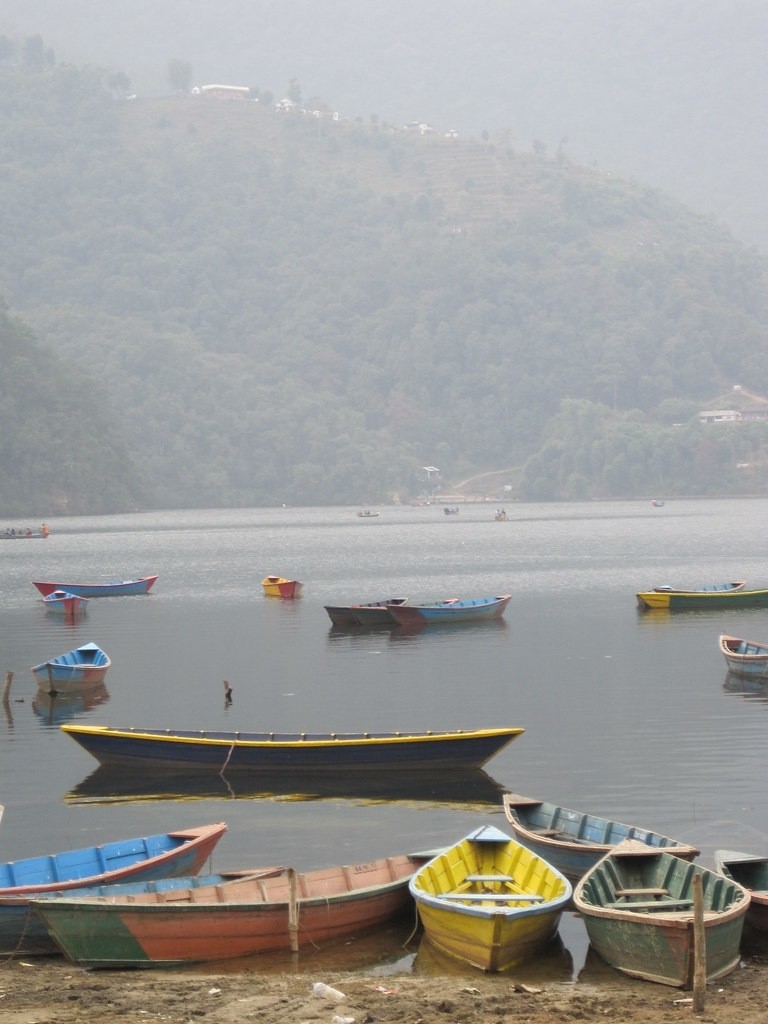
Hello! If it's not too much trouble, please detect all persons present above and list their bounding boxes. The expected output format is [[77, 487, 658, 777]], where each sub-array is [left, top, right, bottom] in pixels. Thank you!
[[357, 510, 370, 515], [3, 523, 48, 535], [443, 505, 459, 512], [495, 507, 505, 518]]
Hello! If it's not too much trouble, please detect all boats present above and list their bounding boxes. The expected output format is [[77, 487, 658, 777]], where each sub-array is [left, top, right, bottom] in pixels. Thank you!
[[325, 594, 406, 628], [352, 596, 458, 628], [444, 508, 458, 514], [357, 509, 380, 518], [261, 575, 302, 598], [1, 819, 225, 956], [5, 527, 50, 539], [636, 576, 768, 609], [497, 510, 507, 523], [410, 822, 570, 972], [31, 640, 110, 697], [573, 835, 751, 987], [714, 845, 768, 928], [43, 586, 90, 616], [26, 838, 453, 971], [501, 788, 701, 890], [385, 595, 512, 625], [60, 716, 522, 774], [720, 634, 768, 680], [30, 573, 154, 597]]
